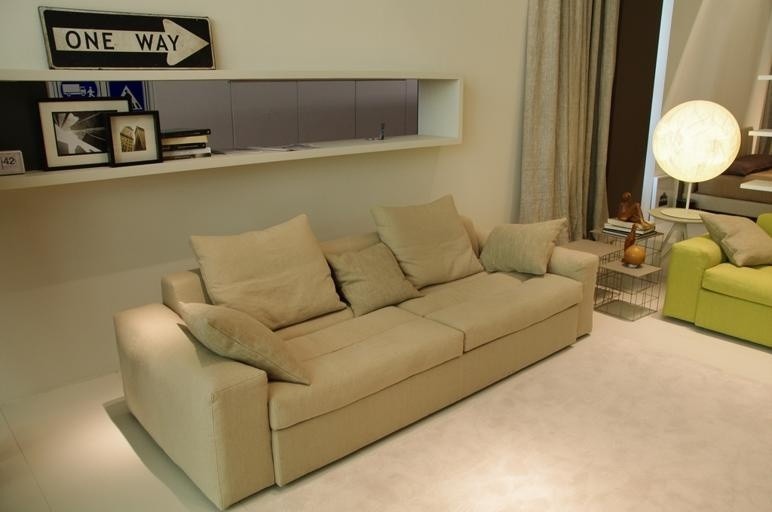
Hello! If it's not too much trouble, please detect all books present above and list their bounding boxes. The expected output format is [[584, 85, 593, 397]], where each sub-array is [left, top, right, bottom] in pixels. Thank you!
[[602, 216, 657, 240], [159, 127, 212, 161], [242, 144, 313, 150]]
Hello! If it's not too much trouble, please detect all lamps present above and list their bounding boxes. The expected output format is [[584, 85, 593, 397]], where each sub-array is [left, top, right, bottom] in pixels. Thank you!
[[651, 100, 742, 219]]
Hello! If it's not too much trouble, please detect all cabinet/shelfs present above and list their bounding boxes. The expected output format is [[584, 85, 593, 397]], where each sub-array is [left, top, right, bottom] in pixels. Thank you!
[[0, 70, 463, 188]]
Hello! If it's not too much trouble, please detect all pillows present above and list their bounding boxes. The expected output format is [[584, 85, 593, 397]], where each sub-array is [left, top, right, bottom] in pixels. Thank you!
[[191, 215, 347, 318], [179, 302, 315, 387], [701, 214, 772, 267], [368, 196, 484, 290], [724, 154, 772, 175], [325, 242, 423, 316], [480, 221, 570, 277]]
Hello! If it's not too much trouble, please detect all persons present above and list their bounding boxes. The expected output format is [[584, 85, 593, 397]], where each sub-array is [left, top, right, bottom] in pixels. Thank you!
[[618, 192, 648, 226]]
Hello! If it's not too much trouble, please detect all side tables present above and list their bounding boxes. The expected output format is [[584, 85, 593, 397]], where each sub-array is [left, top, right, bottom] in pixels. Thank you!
[[649, 207, 704, 264]]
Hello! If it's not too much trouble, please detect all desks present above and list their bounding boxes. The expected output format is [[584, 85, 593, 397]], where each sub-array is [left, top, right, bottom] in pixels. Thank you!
[[564, 226, 664, 320]]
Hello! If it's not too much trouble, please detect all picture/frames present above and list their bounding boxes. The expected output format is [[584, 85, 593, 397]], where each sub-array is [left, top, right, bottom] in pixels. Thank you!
[[651, 176, 678, 223], [104, 110, 163, 167], [34, 96, 131, 171]]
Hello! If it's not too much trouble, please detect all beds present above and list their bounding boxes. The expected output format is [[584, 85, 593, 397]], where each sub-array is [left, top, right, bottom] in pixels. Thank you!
[[684, 127, 772, 218]]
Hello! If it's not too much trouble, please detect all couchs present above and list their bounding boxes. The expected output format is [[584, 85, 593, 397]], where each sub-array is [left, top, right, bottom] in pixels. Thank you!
[[662, 214, 772, 350], [113, 216, 601, 511]]
